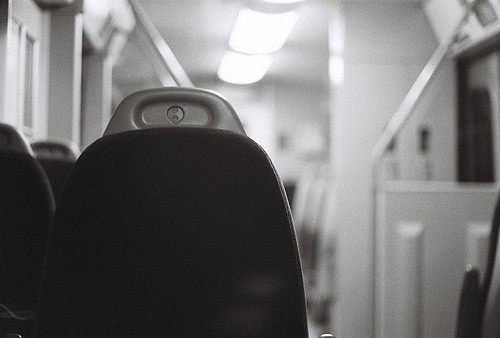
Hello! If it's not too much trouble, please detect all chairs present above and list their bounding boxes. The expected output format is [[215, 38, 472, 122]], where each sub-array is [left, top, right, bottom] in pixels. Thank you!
[[1, 83, 311, 337]]
